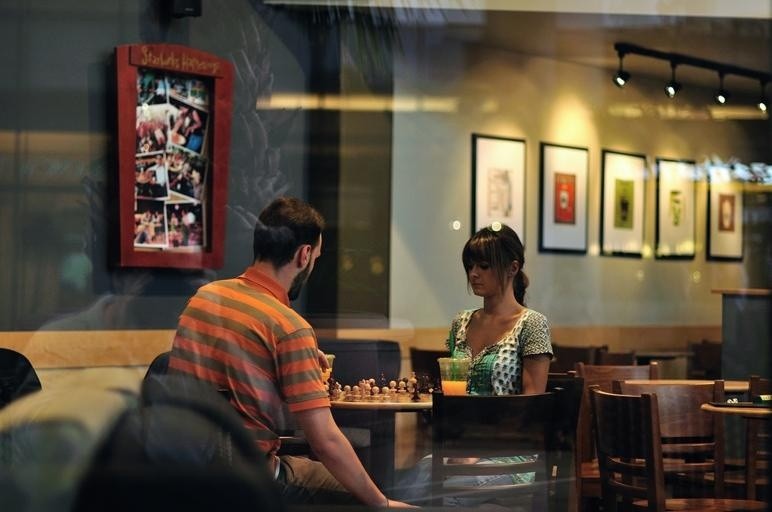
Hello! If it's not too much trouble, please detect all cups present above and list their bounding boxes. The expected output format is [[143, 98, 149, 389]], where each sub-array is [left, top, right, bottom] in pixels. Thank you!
[[321, 354, 336, 382], [438, 358, 470, 397]]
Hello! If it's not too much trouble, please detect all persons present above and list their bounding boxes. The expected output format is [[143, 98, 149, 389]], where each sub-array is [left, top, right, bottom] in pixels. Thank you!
[[134, 70, 211, 250], [403, 224, 554, 511], [168, 197, 421, 508]]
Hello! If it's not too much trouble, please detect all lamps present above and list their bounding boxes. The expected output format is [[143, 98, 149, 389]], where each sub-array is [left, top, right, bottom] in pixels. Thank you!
[[612, 40, 772, 112]]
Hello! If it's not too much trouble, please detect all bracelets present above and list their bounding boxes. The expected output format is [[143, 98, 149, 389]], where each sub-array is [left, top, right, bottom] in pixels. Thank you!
[[387, 498, 389, 508]]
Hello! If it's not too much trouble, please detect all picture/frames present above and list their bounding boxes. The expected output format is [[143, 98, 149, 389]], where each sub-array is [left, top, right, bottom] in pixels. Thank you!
[[470, 133, 526, 253], [706, 162, 743, 261], [601, 148, 646, 259], [114, 42, 235, 271], [537, 143, 588, 254], [656, 158, 697, 261]]
